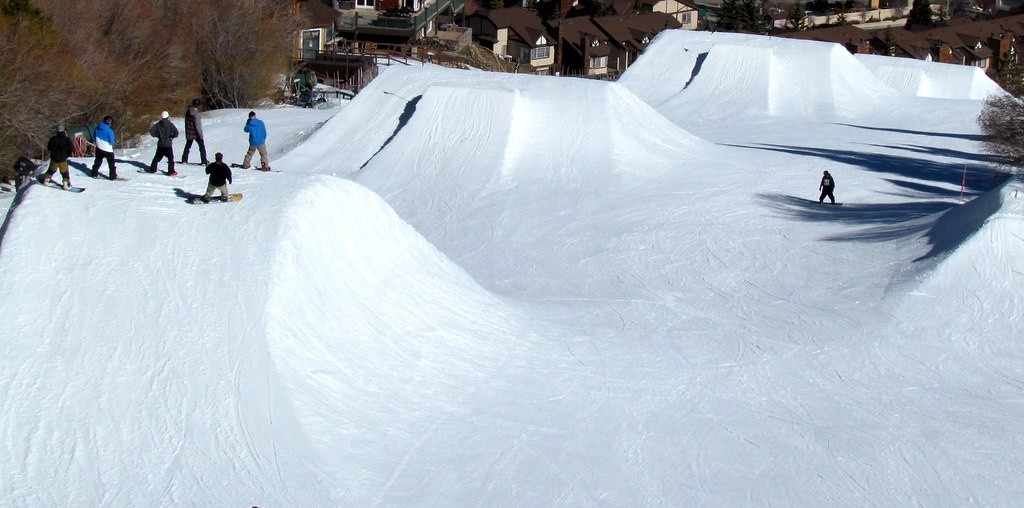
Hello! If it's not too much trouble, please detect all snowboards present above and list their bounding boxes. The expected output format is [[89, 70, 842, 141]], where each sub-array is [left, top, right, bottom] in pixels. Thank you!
[[190, 192, 244, 206], [813, 201, 844, 206], [89, 175, 130, 182], [36, 173, 85, 193], [176, 161, 211, 167], [230, 163, 282, 173], [138, 165, 186, 180]]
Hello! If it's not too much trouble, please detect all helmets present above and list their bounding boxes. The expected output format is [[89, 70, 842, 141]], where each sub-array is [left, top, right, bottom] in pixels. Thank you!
[[104, 116, 113, 125], [193, 99, 201, 106], [56, 124, 64, 132]]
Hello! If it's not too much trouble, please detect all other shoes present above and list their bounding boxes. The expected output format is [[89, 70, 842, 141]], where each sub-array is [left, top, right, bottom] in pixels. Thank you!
[[202, 160, 210, 166], [262, 167, 271, 171], [169, 171, 178, 177], [219, 196, 227, 202], [92, 172, 98, 178], [43, 179, 50, 185], [150, 167, 157, 174], [182, 161, 188, 165], [243, 165, 251, 169], [201, 196, 209, 203], [110, 175, 117, 180], [63, 185, 72, 191]]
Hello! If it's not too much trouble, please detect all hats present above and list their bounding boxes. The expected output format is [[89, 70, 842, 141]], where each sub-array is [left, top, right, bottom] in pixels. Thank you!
[[161, 111, 169, 118]]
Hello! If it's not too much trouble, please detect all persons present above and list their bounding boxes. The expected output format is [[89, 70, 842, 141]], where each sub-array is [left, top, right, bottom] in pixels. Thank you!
[[181, 99, 211, 167], [201, 152, 232, 204], [42, 124, 73, 191], [148, 110, 179, 176], [241, 111, 271, 172], [819, 170, 836, 204], [90, 115, 119, 181]]
